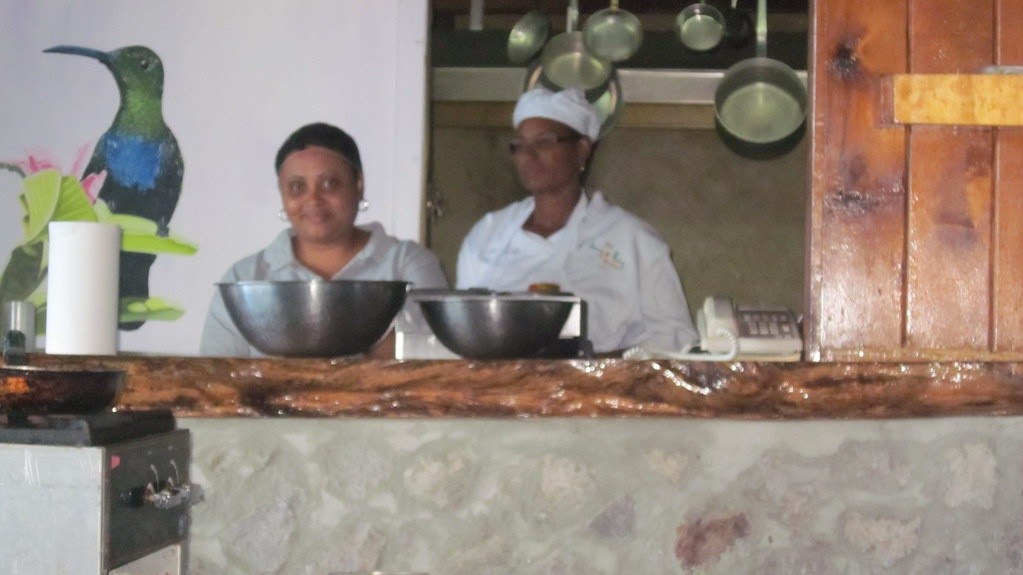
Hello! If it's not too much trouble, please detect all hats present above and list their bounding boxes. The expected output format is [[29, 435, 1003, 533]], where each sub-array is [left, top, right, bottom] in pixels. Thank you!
[[512, 87, 601, 144]]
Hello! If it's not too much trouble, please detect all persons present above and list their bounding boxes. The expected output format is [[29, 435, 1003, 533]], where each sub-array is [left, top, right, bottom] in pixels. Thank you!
[[458, 86, 696, 353], [200, 122, 462, 360]]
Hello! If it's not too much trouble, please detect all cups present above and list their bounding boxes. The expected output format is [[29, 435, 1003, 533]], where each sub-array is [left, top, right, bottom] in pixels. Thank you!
[[45, 220, 122, 357], [3, 300, 36, 353]]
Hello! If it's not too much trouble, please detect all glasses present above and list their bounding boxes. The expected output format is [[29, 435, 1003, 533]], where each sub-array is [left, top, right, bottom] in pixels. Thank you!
[[509, 137, 576, 154]]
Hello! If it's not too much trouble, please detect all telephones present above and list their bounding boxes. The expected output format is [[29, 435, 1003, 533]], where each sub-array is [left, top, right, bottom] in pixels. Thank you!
[[696, 295, 804, 357]]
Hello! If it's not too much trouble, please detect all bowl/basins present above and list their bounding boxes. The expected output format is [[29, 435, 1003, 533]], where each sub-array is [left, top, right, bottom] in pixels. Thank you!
[[214, 280, 413, 359], [410, 288, 581, 359]]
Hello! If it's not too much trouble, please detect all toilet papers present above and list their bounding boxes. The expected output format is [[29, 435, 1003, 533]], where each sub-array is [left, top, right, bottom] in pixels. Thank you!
[[45, 221, 122, 356]]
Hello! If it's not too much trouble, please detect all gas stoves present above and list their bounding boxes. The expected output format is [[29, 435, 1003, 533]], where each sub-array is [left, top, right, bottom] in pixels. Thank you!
[[0, 408, 174, 444]]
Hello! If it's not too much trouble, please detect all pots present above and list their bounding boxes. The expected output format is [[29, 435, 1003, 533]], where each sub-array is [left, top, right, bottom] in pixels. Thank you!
[[518, 0, 625, 140], [0, 363, 127, 415], [583, 0, 643, 63], [673, 0, 727, 53], [722, 0, 755, 45], [506, 9, 549, 66], [712, 0, 809, 161], [541, 0, 614, 102]]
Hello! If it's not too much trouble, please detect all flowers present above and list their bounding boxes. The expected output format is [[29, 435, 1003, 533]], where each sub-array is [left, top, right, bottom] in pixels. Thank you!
[[1, 145, 196, 340]]
